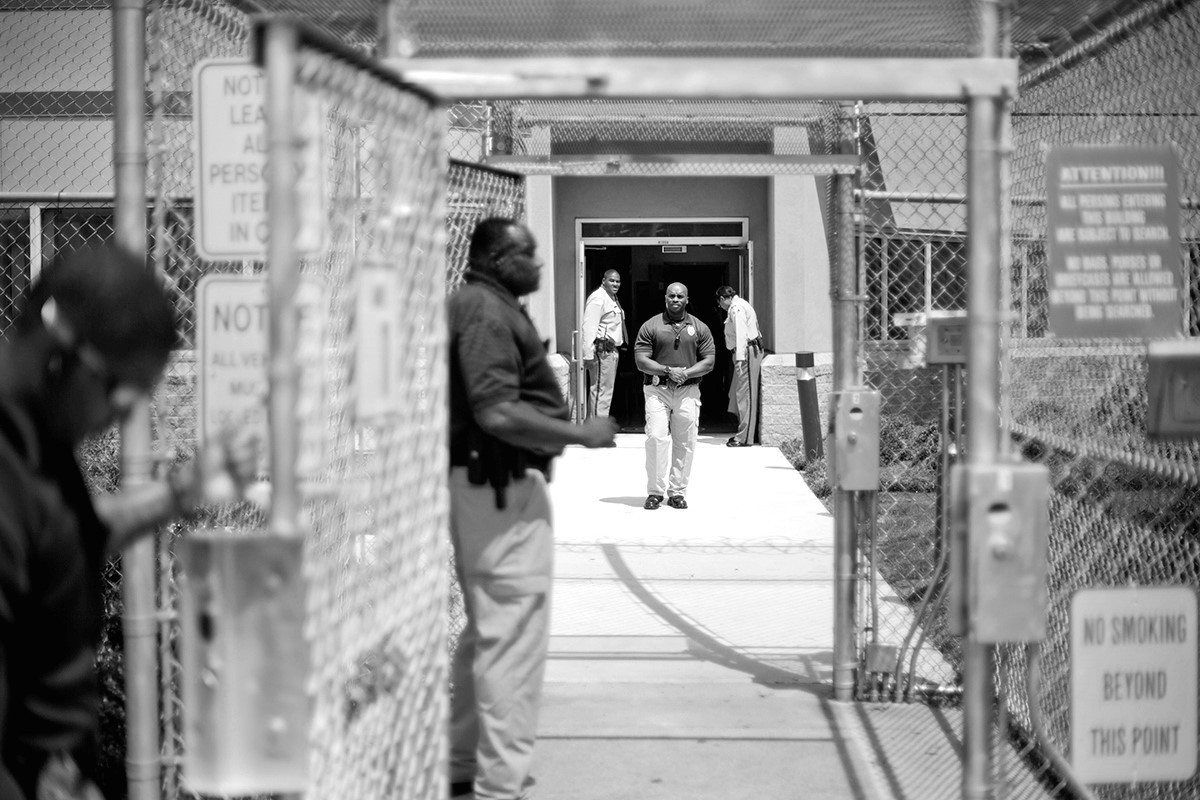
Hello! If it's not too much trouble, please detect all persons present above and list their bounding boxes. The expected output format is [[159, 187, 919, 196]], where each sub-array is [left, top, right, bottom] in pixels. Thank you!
[[582, 269, 629, 449], [715, 285, 765, 447], [634, 282, 717, 510], [446, 215, 619, 800], [0, 240, 256, 800]]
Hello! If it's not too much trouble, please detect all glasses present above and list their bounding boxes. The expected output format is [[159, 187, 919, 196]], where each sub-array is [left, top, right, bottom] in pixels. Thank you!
[[718, 300, 722, 308]]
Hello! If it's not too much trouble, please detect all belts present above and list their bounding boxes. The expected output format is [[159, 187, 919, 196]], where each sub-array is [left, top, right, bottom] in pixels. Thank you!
[[731, 341, 754, 354]]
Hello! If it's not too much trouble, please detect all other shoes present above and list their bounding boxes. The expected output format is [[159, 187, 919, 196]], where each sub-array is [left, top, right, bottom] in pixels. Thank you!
[[452, 769, 534, 795], [727, 438, 745, 447]]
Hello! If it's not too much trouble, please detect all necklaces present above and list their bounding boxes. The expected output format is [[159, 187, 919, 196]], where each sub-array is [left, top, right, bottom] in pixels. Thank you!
[[670, 322, 688, 347]]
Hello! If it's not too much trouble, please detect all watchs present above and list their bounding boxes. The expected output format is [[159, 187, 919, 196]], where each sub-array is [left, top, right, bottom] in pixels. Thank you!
[[664, 365, 670, 376]]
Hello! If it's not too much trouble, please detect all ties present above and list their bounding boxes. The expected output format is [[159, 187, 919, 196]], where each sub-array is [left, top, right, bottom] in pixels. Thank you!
[[614, 295, 629, 345]]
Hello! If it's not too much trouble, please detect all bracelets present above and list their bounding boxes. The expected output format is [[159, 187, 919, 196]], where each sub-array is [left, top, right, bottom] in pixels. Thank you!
[[168, 469, 198, 523]]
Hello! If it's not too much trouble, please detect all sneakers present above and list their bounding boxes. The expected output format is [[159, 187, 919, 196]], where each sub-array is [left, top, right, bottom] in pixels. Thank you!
[[668, 495, 687, 509], [643, 494, 663, 509]]
